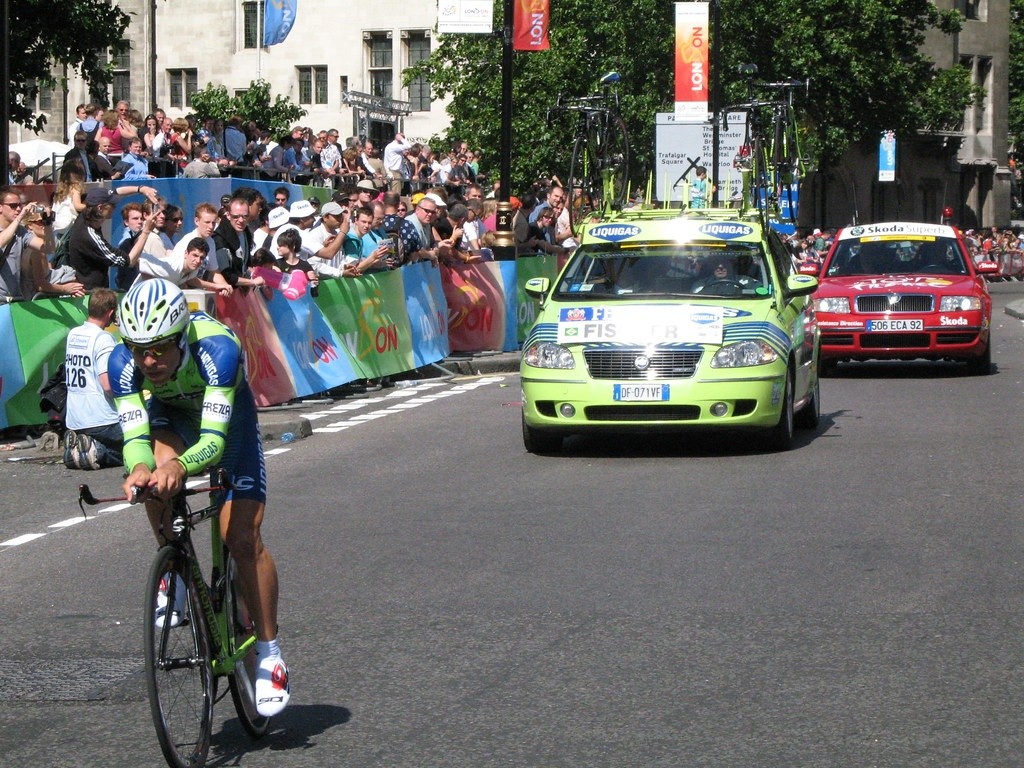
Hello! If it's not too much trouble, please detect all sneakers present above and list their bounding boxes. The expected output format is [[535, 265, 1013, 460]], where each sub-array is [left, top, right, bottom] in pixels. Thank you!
[[63, 430, 81, 470], [76, 434, 100, 471], [154, 571, 187, 629], [255, 659, 290, 717]]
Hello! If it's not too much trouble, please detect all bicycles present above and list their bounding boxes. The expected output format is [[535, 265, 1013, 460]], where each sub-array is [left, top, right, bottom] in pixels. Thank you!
[[77, 467, 271, 768], [725, 67, 811, 234], [545, 71, 630, 240]]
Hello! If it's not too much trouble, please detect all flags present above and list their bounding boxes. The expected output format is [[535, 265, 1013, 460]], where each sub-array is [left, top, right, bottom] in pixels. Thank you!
[[263, 0, 298, 45]]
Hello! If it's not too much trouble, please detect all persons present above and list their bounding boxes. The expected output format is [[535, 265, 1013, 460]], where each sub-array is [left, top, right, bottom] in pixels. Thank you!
[[779, 227, 835, 265], [64, 288, 124, 470], [508, 175, 580, 256], [691, 254, 762, 295], [0, 99, 500, 395], [691, 166, 716, 208], [966, 226, 1024, 252], [106, 278, 292, 717]]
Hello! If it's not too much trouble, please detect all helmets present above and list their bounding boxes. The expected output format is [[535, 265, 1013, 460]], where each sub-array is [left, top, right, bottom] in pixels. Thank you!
[[116, 278, 190, 349]]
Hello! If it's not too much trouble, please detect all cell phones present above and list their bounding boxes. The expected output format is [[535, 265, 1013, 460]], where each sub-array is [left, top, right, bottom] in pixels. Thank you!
[[151, 202, 158, 214]]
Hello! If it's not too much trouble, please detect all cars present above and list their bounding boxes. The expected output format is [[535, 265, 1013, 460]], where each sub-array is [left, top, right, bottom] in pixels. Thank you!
[[518, 221, 823, 457], [797, 221, 1001, 378]]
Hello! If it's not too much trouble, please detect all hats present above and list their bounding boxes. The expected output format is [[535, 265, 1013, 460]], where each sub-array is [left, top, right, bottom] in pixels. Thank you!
[[308, 196, 319, 204], [357, 180, 380, 197], [268, 206, 290, 229], [331, 191, 351, 203], [424, 193, 447, 206], [321, 202, 348, 218], [410, 193, 424, 205], [290, 200, 316, 217], [85, 187, 124, 205]]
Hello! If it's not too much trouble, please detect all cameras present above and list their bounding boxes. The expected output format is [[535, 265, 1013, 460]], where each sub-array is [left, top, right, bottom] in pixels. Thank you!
[[35, 204, 45, 214], [161, 143, 174, 156]]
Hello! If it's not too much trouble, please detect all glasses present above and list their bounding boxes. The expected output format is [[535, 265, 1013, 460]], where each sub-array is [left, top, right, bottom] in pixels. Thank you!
[[167, 217, 183, 222], [716, 267, 727, 272], [118, 109, 128, 111], [543, 214, 554, 220], [470, 209, 478, 217], [230, 214, 249, 219], [363, 189, 374, 195], [122, 334, 177, 358], [460, 158, 466, 162], [397, 209, 405, 212], [3, 203, 22, 208], [419, 206, 435, 214], [276, 198, 285, 202]]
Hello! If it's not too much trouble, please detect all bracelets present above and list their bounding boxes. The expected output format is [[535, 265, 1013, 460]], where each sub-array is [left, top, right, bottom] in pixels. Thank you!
[[340, 230, 346, 236], [137, 185, 143, 192], [464, 256, 469, 264]]
